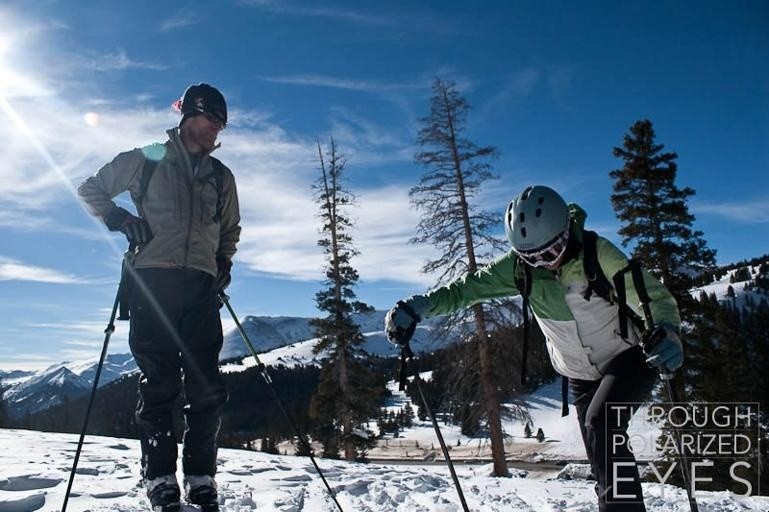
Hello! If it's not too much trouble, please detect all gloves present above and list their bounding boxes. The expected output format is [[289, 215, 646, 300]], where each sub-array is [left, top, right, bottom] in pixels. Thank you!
[[639, 325, 685, 373], [103, 205, 153, 245], [213, 254, 233, 294], [384, 300, 421, 346]]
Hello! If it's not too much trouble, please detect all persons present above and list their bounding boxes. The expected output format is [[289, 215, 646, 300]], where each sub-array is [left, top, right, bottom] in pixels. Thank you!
[[383, 183, 685, 512], [75, 80, 242, 512]]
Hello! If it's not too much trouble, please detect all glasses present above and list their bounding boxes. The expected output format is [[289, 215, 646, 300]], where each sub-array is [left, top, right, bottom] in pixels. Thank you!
[[511, 214, 571, 269]]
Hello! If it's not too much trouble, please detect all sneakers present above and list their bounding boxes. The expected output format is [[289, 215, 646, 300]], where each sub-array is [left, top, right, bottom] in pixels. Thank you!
[[183, 474, 218, 505], [144, 473, 180, 508]]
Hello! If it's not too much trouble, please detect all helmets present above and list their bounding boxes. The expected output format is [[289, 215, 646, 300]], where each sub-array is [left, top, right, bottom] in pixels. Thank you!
[[504, 184, 569, 251]]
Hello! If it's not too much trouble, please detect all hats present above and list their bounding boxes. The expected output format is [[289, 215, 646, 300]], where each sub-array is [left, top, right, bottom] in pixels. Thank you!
[[182, 81, 228, 123]]
